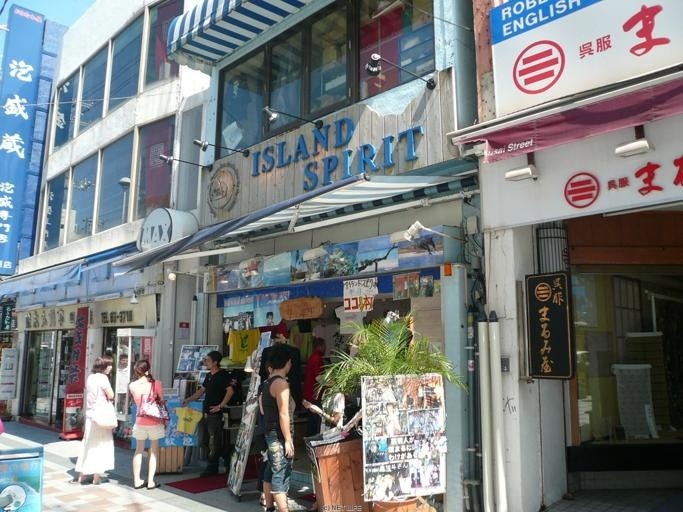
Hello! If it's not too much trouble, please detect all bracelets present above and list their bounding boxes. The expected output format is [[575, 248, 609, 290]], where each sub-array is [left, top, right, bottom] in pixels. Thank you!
[[218, 403, 223, 410]]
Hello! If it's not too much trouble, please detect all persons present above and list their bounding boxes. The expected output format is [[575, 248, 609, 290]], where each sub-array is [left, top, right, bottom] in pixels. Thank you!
[[300, 368, 345, 435], [180, 349, 237, 479], [255, 347, 298, 506], [302, 336, 326, 404], [127, 360, 170, 489], [258, 347, 294, 511], [73, 354, 117, 485], [366, 440, 385, 463], [264, 311, 275, 326], [385, 404, 401, 437], [116, 354, 127, 413]]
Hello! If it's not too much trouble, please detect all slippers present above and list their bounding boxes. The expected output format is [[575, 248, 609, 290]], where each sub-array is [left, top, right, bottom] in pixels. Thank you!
[[133, 478, 161, 489]]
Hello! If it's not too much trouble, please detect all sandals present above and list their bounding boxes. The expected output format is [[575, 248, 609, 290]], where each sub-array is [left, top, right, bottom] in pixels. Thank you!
[[75, 475, 110, 486]]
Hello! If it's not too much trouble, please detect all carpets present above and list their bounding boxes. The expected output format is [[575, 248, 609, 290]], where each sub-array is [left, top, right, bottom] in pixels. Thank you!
[[164, 450, 298, 494]]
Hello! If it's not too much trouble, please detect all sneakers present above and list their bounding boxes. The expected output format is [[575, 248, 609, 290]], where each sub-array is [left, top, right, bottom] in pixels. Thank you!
[[198, 462, 231, 477]]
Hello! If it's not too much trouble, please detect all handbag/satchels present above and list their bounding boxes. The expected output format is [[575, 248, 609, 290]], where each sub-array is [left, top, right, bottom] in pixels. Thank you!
[[90, 371, 119, 430], [135, 377, 171, 422]]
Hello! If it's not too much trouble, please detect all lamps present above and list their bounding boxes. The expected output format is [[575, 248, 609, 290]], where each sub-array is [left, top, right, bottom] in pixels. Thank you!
[[165, 267, 195, 282], [157, 137, 250, 173], [364, 53, 436, 91], [402, 219, 461, 248], [261, 105, 323, 130]]
